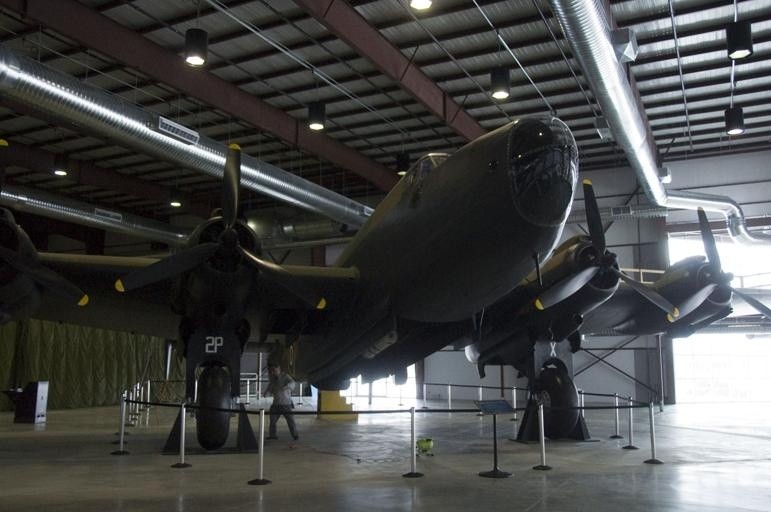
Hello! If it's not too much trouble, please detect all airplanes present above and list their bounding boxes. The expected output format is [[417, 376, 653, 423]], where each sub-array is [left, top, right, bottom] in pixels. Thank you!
[[0, 108, 771, 450]]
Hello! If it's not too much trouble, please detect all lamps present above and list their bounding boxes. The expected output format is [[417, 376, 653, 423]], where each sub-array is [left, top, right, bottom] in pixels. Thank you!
[[724, 104, 745, 135], [395, 134, 409, 177], [184, 1, 210, 67], [490, 37, 511, 100], [725, 20, 754, 61], [308, 78, 326, 131]]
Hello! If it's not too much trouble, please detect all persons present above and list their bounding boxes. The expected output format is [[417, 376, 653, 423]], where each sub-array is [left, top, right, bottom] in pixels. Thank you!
[[264, 362, 299, 441]]
